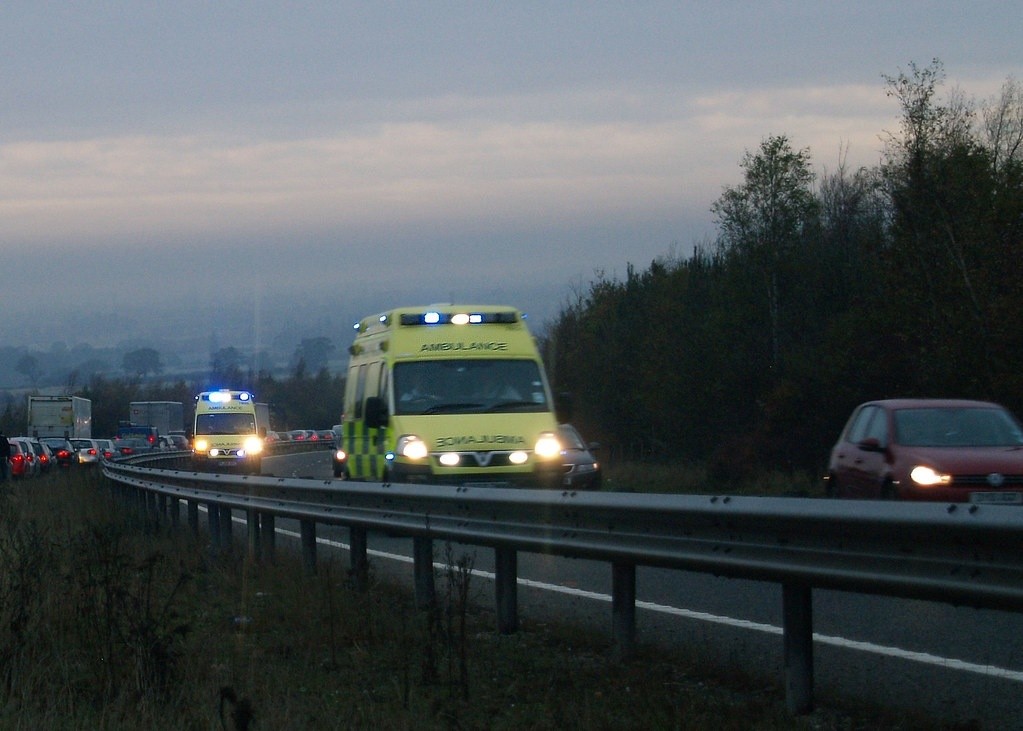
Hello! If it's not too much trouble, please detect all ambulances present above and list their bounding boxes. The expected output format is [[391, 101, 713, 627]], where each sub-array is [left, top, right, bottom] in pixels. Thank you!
[[184, 387, 268, 477], [336, 302, 563, 491]]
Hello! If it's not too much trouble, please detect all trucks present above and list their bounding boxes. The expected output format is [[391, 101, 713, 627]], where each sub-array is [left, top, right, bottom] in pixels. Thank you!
[[118, 400, 187, 451], [27, 394, 92, 441]]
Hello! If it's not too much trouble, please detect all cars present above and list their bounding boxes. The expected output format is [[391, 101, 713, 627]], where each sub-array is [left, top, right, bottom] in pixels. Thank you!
[[328, 433, 348, 479], [0, 433, 193, 480], [820, 398, 1023, 506], [558, 420, 603, 491], [259, 424, 344, 443]]
